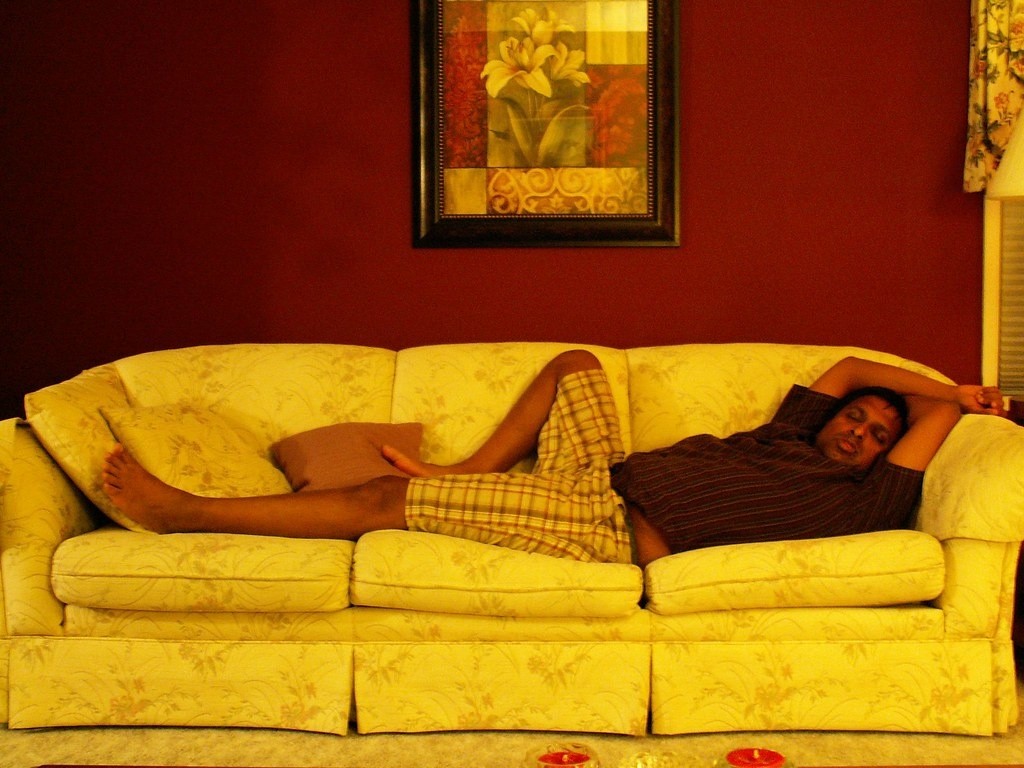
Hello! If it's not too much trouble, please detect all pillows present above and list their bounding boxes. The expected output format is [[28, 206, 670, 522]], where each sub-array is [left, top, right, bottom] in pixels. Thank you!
[[99, 400, 295, 499], [22, 362, 160, 537], [264, 421, 424, 495]]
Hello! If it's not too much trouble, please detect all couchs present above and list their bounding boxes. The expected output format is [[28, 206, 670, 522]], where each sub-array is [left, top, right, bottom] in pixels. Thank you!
[[0, 343, 1024, 739]]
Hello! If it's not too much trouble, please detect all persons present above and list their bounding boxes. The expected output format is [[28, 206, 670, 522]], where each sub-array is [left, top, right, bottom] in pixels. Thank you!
[[99, 349, 1005, 566]]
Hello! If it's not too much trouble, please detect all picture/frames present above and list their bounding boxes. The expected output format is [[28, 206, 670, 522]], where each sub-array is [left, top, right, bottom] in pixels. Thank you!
[[409, 0, 680, 249]]
